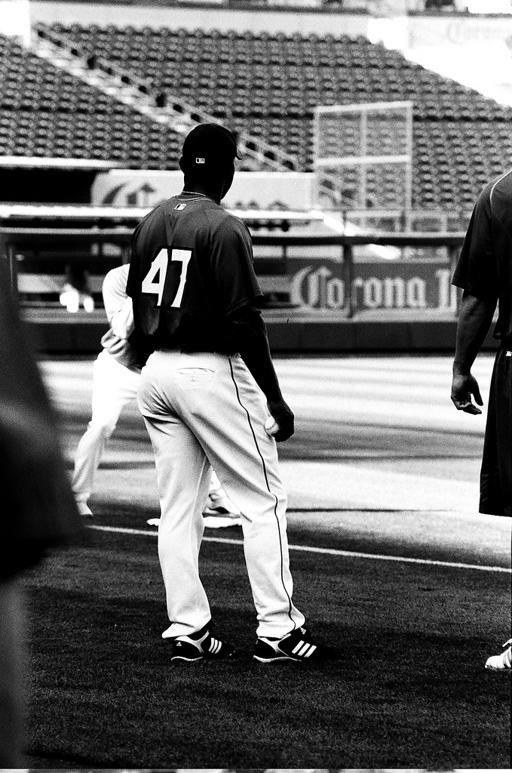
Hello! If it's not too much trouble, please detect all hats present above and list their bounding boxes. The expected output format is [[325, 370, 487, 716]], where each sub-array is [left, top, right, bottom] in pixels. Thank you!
[[179, 121, 238, 170]]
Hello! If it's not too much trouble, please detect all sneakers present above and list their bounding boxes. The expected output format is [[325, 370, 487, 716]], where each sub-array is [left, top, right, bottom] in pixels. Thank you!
[[76, 501, 94, 519], [252, 625, 323, 663], [171, 628, 237, 664], [483, 638, 512, 673], [209, 492, 235, 516]]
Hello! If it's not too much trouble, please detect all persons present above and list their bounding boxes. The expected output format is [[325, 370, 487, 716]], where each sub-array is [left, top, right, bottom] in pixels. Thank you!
[[451, 169, 512, 670], [126, 123, 340, 665], [71, 264, 241, 517]]
